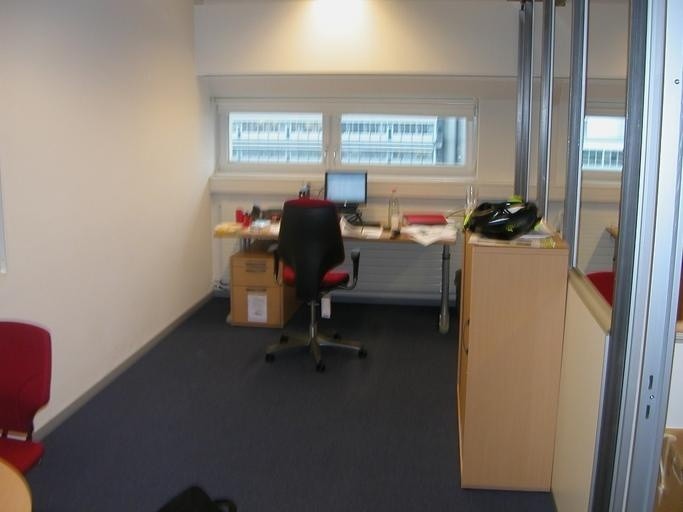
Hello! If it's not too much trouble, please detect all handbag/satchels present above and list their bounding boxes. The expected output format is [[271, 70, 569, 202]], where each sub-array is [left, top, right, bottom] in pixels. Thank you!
[[465, 201, 542, 241]]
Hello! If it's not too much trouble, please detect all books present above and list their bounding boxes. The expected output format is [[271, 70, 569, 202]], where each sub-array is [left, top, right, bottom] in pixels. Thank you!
[[400, 214, 458, 247]]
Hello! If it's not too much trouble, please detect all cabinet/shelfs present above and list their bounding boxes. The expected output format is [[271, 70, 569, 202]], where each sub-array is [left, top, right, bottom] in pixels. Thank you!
[[229, 240, 303, 329], [456, 219, 571, 493]]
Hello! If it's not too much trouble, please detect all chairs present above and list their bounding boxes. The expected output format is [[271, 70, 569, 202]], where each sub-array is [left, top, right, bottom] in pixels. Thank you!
[[656, 320, 683, 511], [0, 321, 52, 475], [263, 199, 368, 372]]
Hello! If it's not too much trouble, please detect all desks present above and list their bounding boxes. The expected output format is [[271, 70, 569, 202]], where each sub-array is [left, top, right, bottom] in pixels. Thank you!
[[0, 457, 34, 512], [212, 221, 455, 336]]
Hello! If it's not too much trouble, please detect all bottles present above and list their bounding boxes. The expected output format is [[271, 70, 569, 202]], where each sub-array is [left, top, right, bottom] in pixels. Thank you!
[[387, 188, 402, 237]]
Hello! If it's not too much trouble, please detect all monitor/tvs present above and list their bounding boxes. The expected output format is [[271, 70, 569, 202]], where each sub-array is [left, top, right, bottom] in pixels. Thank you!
[[324, 170, 368, 206]]
